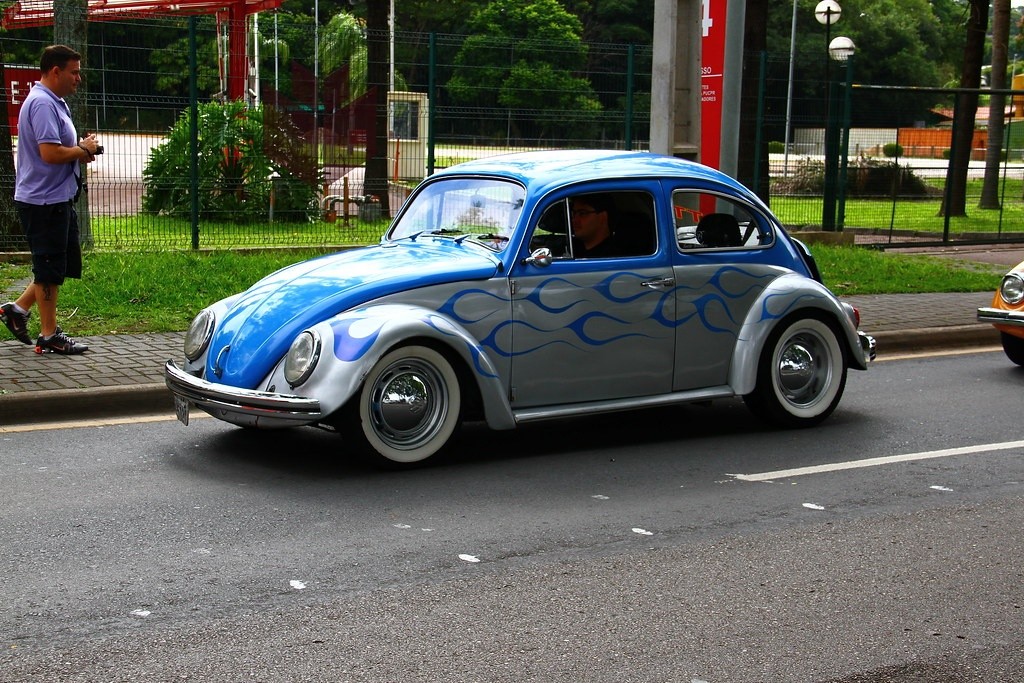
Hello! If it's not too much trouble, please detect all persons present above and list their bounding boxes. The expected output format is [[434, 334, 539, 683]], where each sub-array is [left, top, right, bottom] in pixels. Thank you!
[[0, 45, 100, 355], [532, 195, 623, 258]]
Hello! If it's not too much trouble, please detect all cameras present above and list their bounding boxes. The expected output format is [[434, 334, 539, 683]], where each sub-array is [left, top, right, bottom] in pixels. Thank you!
[[91, 146, 103, 155]]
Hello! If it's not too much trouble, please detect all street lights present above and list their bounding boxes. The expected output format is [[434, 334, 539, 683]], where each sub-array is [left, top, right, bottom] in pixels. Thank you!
[[813, 0, 856, 230]]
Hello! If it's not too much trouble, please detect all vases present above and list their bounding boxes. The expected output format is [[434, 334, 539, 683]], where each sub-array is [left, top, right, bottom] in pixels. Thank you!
[[321, 210, 337, 224]]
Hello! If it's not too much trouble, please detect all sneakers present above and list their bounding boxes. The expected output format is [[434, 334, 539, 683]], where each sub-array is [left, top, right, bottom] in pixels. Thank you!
[[36, 327, 88, 355], [0, 302, 32, 345]]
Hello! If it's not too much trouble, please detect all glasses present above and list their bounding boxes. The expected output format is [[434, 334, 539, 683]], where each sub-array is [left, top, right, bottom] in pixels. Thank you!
[[571, 209, 597, 217]]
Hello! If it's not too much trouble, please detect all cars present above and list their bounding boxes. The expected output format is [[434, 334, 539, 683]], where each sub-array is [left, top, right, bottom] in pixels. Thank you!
[[162, 148, 878, 467], [976, 253, 1024, 366]]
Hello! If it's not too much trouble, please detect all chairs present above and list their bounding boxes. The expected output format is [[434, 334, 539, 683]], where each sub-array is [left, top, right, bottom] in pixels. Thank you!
[[696, 212, 744, 248], [529, 202, 575, 257]]
